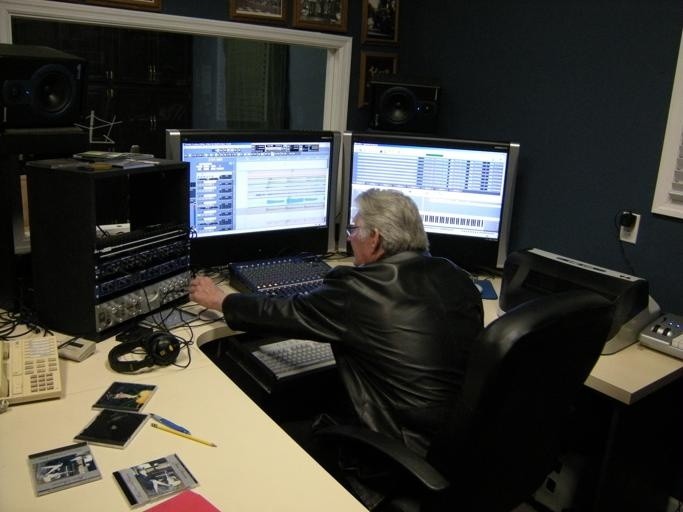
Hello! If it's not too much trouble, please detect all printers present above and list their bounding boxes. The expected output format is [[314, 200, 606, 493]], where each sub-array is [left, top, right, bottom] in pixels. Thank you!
[[496, 246, 661, 355]]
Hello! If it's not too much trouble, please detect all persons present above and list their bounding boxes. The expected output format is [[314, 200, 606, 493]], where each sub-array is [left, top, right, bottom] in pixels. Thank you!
[[187, 188, 486, 493], [136, 466, 171, 495], [41, 455, 83, 476]]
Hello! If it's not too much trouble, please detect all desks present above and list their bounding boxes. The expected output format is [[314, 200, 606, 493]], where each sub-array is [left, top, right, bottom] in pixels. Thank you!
[[0, 252, 683, 512]]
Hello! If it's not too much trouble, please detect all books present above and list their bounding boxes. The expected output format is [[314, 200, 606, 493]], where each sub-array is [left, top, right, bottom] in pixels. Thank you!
[[27, 442, 103, 497], [111, 452, 200, 509], [91, 381, 159, 413], [73, 409, 151, 450]]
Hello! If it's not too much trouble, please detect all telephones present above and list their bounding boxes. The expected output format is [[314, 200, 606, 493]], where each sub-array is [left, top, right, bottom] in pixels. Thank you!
[[0, 335, 63, 413]]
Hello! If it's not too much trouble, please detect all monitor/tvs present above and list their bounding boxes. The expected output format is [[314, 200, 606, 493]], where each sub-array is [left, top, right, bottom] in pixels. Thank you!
[[166, 129, 337, 256], [338, 131, 521, 271]]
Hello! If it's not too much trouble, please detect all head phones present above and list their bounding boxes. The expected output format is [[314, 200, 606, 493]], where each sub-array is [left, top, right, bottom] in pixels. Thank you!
[[108, 330, 181, 374]]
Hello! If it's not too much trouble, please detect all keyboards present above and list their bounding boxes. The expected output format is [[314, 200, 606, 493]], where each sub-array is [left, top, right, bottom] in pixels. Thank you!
[[252, 338, 336, 381]]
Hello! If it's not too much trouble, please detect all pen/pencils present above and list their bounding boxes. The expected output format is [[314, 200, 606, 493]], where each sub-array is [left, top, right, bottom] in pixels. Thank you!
[[150, 412, 190, 435], [151, 423, 217, 447]]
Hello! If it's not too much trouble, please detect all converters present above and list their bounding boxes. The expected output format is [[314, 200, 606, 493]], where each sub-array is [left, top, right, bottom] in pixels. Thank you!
[[618, 213, 636, 229]]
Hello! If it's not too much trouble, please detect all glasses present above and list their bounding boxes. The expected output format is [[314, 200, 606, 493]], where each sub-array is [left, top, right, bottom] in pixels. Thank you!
[[345, 224, 360, 236]]
